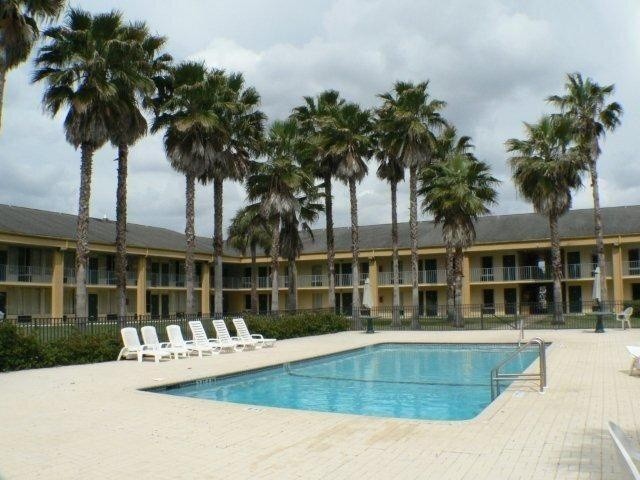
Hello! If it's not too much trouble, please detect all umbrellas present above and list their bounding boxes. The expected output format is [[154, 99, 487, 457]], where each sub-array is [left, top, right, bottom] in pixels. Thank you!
[[361, 276, 374, 315], [590, 266, 604, 310]]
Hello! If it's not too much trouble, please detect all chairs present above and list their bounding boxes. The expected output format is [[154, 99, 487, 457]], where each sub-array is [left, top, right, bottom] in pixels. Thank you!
[[607, 420, 640, 480], [114, 318, 277, 369], [616, 303, 633, 330]]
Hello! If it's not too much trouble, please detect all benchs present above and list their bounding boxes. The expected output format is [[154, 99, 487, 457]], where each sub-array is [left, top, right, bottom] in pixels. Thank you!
[[626, 345, 640, 376]]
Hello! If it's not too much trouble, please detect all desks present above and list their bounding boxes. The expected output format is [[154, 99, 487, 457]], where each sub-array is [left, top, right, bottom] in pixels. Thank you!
[[585, 310, 617, 334], [358, 316, 382, 334]]
